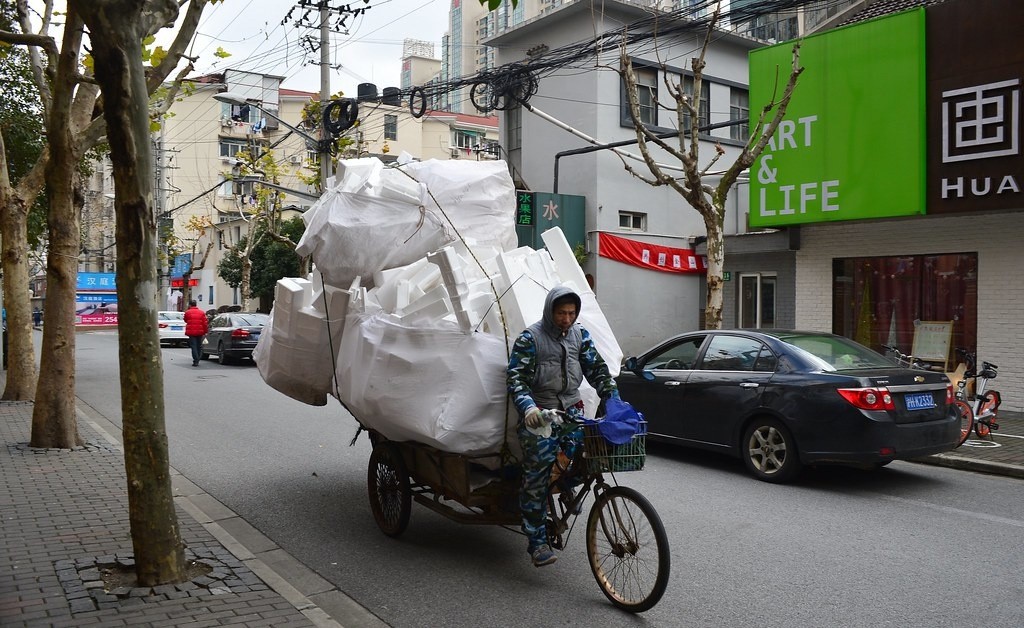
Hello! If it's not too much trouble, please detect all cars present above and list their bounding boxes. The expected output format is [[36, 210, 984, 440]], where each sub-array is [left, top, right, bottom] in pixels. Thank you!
[[157, 310, 192, 347], [197, 312, 270, 365], [610, 326, 963, 485]]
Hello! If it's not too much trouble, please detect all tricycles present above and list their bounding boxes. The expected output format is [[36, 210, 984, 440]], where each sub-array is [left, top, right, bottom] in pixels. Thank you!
[[367, 403, 671, 614]]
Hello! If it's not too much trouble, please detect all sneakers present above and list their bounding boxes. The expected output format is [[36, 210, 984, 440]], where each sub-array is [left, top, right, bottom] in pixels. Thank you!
[[560, 485, 583, 515], [531, 543, 558, 565]]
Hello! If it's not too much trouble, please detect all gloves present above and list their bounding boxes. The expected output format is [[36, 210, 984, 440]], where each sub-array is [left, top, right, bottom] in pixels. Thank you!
[[525, 408, 545, 429]]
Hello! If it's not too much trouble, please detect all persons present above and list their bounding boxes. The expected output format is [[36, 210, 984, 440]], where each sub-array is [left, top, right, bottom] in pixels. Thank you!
[[2, 308, 7, 323], [33, 307, 44, 326], [183, 300, 209, 365], [505, 286, 622, 566]]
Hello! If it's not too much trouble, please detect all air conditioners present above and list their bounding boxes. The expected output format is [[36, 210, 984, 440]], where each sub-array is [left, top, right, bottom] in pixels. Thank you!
[[222, 119, 234, 127], [290, 155, 302, 162]]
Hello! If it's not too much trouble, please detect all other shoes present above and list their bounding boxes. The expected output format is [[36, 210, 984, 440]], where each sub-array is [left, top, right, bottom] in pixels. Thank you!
[[192, 362, 199, 366]]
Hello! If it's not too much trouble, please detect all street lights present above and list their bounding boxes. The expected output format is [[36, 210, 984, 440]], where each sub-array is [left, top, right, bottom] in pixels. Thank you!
[[210, 90, 332, 203]]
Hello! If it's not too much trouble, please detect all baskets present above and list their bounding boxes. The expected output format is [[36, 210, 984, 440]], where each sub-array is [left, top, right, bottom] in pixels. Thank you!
[[893, 357, 924, 369], [578, 421, 648, 472]]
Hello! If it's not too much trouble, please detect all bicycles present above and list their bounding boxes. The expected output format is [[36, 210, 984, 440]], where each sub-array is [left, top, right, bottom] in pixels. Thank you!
[[881, 341, 1003, 448]]
